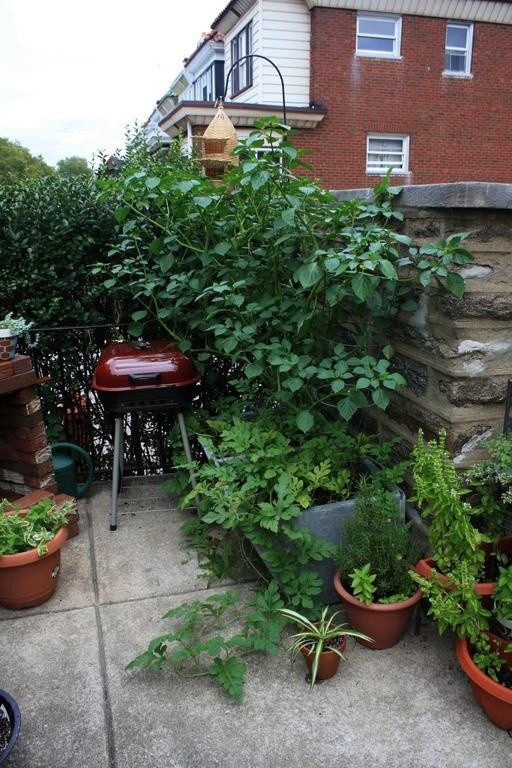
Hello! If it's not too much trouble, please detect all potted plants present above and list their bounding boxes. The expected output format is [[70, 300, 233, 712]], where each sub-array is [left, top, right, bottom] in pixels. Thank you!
[[0, 309, 39, 362], [0, 494, 80, 613], [124, 394, 511, 733]]
